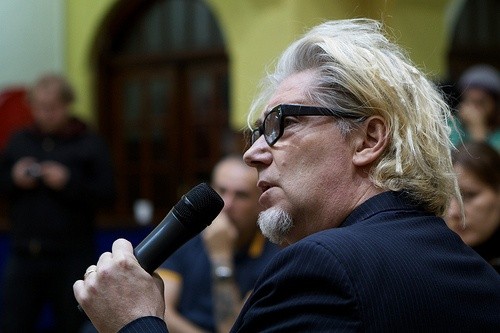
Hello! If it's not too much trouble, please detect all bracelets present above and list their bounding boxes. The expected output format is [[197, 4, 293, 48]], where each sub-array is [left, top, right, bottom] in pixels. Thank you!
[[210, 266, 237, 281]]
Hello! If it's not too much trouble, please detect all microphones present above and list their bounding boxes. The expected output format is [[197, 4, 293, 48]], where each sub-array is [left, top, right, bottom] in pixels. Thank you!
[[132, 183, 225, 276]]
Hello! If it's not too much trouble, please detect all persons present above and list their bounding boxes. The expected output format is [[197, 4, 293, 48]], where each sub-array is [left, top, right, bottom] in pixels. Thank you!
[[446, 66, 500, 153], [72, 16, 499, 333], [442, 139, 500, 277], [0, 72, 99, 333], [149, 151, 280, 333]]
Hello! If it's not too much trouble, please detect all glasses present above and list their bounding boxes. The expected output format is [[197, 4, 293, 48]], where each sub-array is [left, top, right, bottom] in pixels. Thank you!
[[251, 104, 363, 147]]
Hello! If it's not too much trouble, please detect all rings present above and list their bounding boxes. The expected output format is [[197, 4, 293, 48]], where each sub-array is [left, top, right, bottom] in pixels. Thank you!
[[84, 270, 95, 278]]
